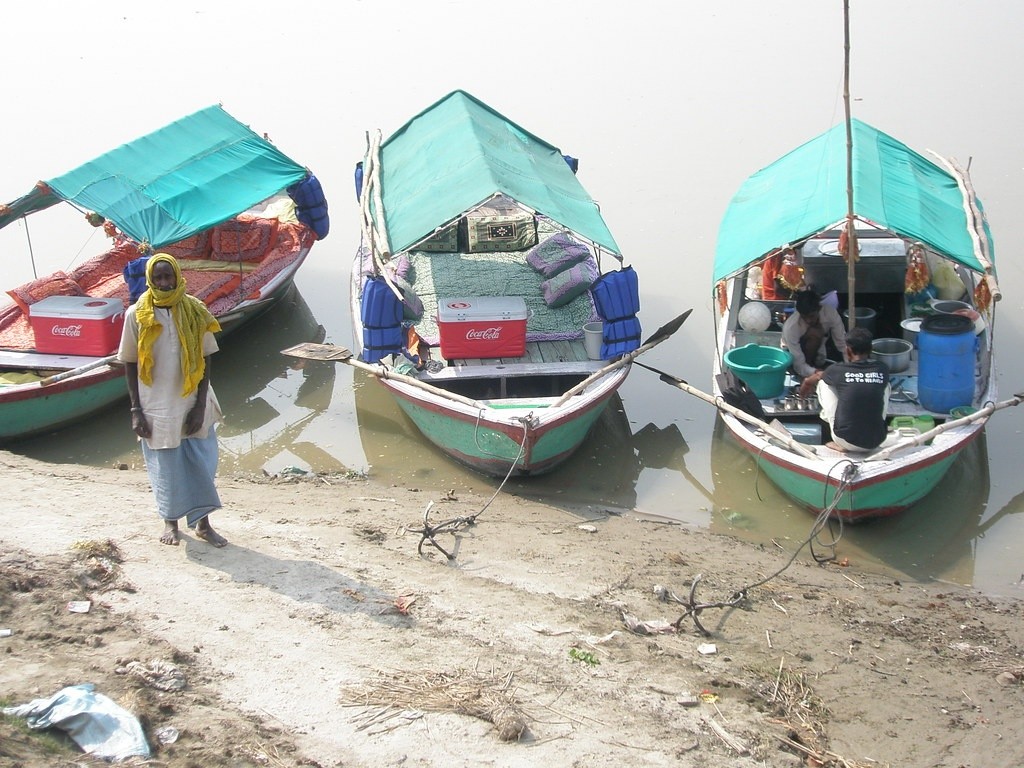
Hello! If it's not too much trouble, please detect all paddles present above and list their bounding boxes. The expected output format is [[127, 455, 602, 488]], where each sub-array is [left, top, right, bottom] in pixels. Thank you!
[[628, 421, 720, 508], [280, 342, 496, 412], [547, 308, 693, 407], [863, 392, 1024, 463], [631, 360, 824, 462], [968, 488, 1024, 539]]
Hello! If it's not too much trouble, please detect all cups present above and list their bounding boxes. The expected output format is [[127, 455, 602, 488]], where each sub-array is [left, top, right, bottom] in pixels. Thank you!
[[783, 392, 819, 410]]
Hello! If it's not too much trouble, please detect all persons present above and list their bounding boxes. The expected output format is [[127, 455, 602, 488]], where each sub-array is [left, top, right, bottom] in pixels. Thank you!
[[778, 249, 808, 295], [762, 247, 790, 301], [117, 253, 228, 548], [745, 258, 762, 301], [780, 290, 849, 379], [799, 326, 891, 453]]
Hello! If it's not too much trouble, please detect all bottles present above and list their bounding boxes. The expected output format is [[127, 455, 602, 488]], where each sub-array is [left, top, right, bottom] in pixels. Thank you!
[[890, 414, 935, 445]]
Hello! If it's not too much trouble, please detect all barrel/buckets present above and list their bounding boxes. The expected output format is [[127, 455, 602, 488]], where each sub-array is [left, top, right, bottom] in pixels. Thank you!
[[582, 321, 605, 359], [724, 342, 794, 398], [844, 308, 877, 336], [918, 314, 975, 413]]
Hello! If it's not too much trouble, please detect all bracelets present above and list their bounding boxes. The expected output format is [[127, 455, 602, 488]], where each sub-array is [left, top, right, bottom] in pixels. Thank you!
[[130, 406, 142, 413]]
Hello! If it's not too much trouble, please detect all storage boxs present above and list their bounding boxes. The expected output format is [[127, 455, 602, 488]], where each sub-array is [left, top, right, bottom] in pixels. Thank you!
[[800, 239, 906, 294], [436, 295, 529, 359], [29, 295, 124, 357]]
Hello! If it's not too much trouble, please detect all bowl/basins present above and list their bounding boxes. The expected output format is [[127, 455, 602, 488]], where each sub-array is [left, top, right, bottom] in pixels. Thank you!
[[817, 290, 840, 309], [931, 300, 974, 313], [901, 317, 924, 350], [869, 338, 914, 372]]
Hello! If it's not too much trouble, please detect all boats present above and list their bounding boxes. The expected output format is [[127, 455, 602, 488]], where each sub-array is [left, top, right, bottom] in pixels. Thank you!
[[351, 87, 641, 481], [713, 118, 1002, 528], [1, 99, 332, 447]]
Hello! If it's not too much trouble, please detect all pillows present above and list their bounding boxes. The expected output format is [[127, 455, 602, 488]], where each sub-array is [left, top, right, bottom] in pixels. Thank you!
[[153, 228, 213, 263], [526, 233, 591, 278], [386, 271, 423, 320], [388, 254, 412, 279], [6, 269, 84, 319], [543, 261, 591, 308], [464, 212, 538, 254], [212, 215, 280, 263], [403, 214, 461, 254]]
